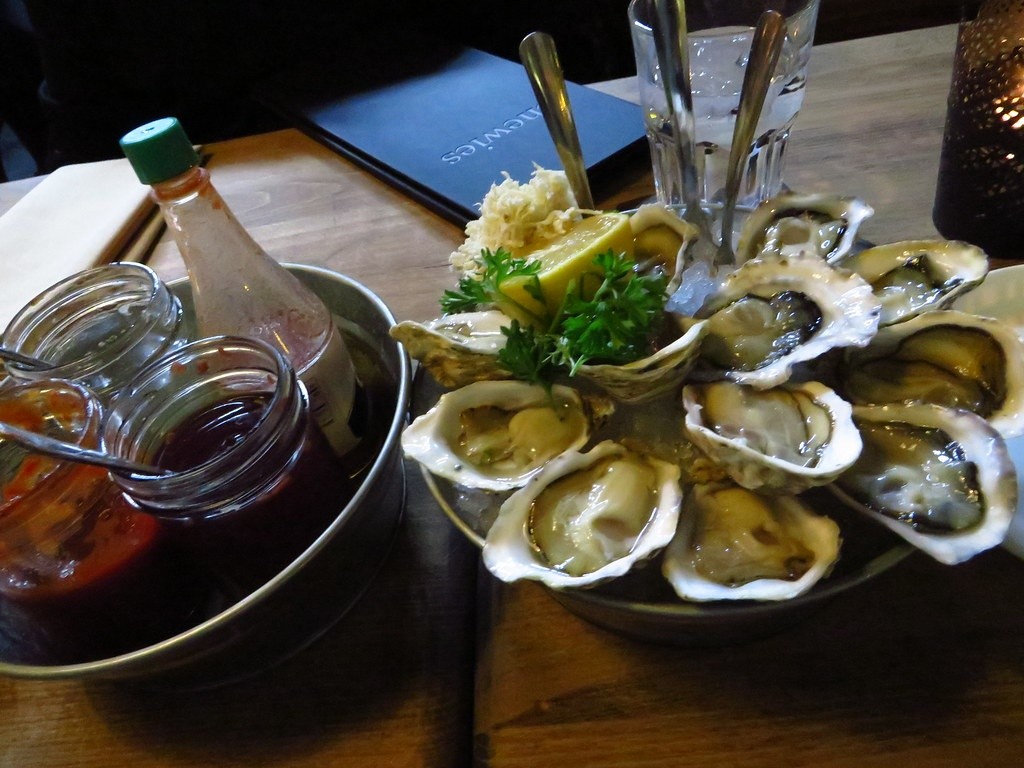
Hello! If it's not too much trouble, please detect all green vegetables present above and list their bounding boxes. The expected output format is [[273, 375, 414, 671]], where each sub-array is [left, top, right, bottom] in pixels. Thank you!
[[440, 244, 674, 407]]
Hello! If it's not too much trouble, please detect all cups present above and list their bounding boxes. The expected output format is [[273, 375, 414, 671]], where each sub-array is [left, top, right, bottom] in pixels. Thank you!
[[103, 336, 333, 581], [0, 385, 156, 608], [626, 0, 817, 216], [931, 0, 1024, 258], [6, 262, 187, 405]]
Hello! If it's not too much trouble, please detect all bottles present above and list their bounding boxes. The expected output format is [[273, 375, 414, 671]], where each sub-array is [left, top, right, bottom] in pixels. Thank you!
[[122, 118, 369, 495]]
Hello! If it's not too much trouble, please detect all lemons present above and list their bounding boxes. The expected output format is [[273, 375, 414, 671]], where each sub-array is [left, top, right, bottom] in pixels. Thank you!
[[499, 213, 636, 316]]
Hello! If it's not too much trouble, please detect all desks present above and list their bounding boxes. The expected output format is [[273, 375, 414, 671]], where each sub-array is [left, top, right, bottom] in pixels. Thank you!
[[0, 24, 1024, 768]]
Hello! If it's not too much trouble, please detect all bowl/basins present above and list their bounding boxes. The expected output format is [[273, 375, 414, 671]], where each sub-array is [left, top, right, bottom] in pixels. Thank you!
[[410, 210, 922, 639], [0, 267, 405, 676]]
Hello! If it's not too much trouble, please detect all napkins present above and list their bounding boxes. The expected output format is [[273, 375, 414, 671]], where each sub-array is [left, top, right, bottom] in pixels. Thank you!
[[1, 144, 202, 350]]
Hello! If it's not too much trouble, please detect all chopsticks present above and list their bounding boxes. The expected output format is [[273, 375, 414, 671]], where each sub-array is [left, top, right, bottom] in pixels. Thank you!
[[96, 142, 210, 264]]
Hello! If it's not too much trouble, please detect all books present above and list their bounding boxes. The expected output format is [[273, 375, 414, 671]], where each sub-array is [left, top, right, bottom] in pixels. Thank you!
[[294, 45, 652, 241]]
